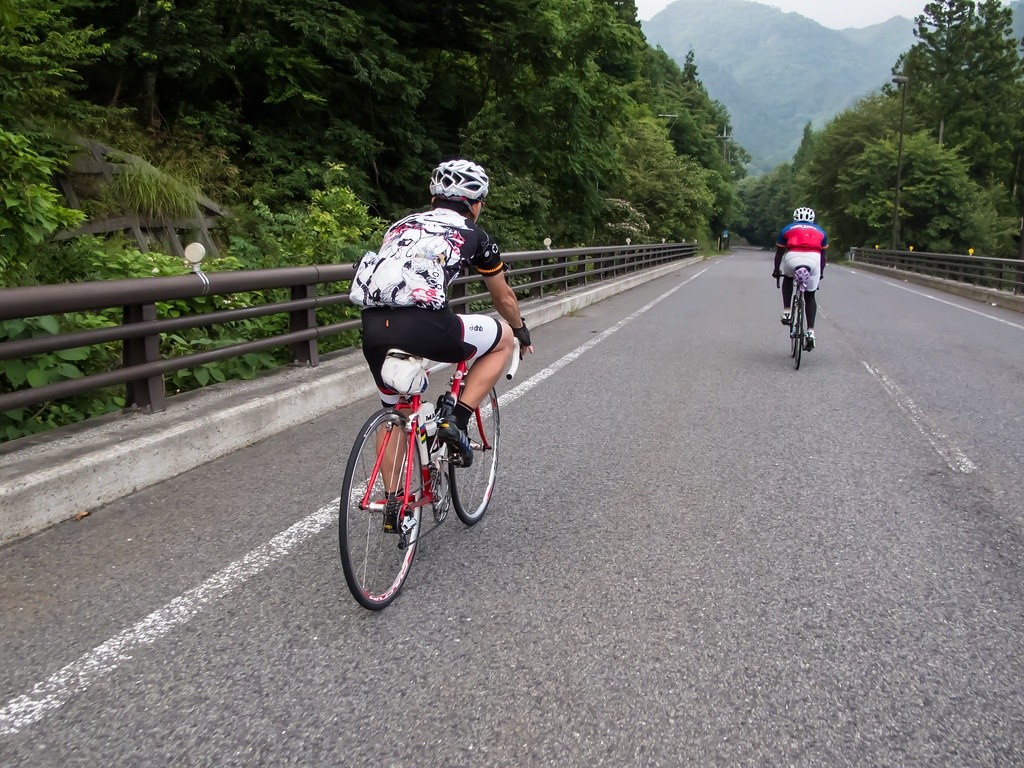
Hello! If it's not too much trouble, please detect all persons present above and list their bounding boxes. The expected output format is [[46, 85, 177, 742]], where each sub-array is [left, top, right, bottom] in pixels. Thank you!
[[349, 158, 534, 534], [772, 207, 828, 351]]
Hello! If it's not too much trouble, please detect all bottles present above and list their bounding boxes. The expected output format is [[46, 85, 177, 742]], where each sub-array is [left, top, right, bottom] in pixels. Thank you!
[[436, 391, 455, 447], [418, 400, 440, 459]]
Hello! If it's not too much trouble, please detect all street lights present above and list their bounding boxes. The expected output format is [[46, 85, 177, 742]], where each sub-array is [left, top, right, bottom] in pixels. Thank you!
[[892, 76, 908, 248]]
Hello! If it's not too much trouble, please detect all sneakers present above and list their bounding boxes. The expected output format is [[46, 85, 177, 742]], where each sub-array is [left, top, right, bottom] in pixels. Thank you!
[[781, 312, 792, 325], [805, 330, 816, 349], [437, 414, 474, 467], [382, 512, 406, 533]]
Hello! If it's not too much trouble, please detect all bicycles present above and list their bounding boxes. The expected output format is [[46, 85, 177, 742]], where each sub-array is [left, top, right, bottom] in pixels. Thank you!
[[337, 336, 523, 611], [771, 264, 824, 371]]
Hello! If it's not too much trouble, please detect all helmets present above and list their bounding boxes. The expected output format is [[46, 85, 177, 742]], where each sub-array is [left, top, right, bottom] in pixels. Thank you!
[[792, 206, 816, 224], [428, 159, 490, 214]]
